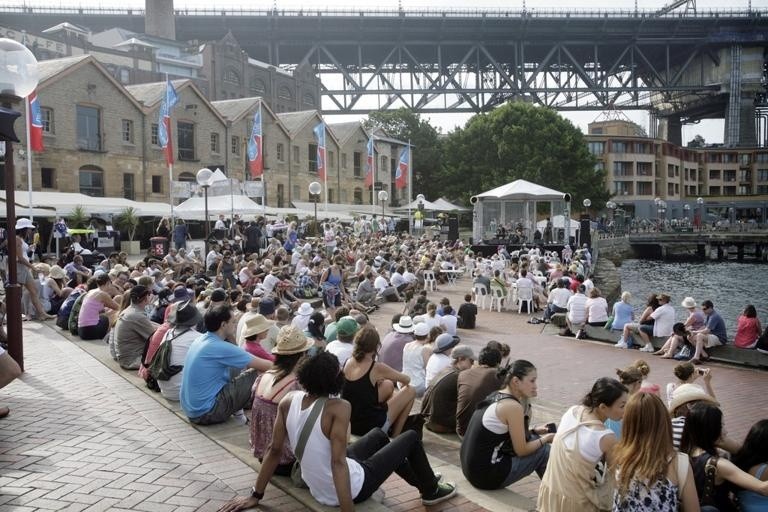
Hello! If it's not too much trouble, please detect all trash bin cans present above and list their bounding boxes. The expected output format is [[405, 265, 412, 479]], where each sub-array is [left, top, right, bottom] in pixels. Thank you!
[[149, 236, 169, 258]]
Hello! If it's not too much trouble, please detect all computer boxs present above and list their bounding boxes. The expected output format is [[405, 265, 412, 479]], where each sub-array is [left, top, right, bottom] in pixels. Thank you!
[[554, 227, 565, 241]]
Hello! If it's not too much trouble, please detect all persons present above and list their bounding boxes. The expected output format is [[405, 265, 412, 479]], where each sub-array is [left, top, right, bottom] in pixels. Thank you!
[[1, 213, 766, 510]]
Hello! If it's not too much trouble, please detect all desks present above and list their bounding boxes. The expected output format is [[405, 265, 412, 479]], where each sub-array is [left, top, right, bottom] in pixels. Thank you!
[[440, 269, 464, 286]]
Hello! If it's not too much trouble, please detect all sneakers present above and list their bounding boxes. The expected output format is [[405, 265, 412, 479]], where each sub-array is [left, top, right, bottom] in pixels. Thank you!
[[430, 472, 442, 480], [614, 341, 628, 349], [0, 405, 10, 420], [700, 354, 710, 362], [420, 480, 460, 507], [686, 359, 703, 367], [638, 343, 655, 353]]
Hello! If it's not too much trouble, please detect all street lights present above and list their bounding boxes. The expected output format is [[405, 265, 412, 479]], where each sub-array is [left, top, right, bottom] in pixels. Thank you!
[[605, 196, 709, 230], [377, 190, 390, 235], [307, 181, 322, 238], [582, 199, 591, 216], [195, 167, 215, 241], [0, 37, 40, 371], [415, 194, 425, 236]]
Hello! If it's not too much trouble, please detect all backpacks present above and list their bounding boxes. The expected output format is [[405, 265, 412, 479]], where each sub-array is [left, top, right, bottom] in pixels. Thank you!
[[699, 491, 743, 512], [147, 340, 183, 381]]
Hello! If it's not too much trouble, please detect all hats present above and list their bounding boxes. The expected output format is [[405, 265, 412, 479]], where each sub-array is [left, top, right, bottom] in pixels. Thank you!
[[656, 293, 672, 301], [130, 284, 148, 303], [270, 323, 315, 356], [269, 267, 281, 275], [258, 298, 276, 315], [167, 285, 194, 302], [391, 315, 415, 333], [245, 296, 261, 311], [682, 297, 697, 309], [240, 314, 275, 340], [15, 218, 36, 230], [211, 287, 227, 302], [335, 317, 360, 338], [48, 265, 67, 279], [297, 302, 315, 315], [431, 333, 461, 354], [450, 344, 478, 363], [166, 299, 207, 328], [164, 269, 175, 275], [109, 264, 130, 275], [413, 322, 429, 337], [252, 289, 263, 296], [667, 382, 720, 417]]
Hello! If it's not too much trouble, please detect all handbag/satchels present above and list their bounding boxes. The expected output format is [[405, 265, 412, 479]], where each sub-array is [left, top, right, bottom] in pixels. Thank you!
[[674, 345, 694, 361], [290, 461, 307, 489], [603, 316, 615, 331]]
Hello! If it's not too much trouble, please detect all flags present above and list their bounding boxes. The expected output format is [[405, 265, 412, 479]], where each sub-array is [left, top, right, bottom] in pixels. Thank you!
[[28, 91, 46, 152], [313, 122, 327, 183], [247, 108, 264, 178], [395, 145, 410, 190], [365, 135, 373, 187], [158, 80, 179, 166]]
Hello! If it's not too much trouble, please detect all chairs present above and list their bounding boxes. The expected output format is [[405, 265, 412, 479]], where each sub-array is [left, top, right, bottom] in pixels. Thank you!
[[423, 270, 438, 293], [534, 276, 547, 286], [474, 282, 492, 310], [518, 287, 534, 315], [489, 285, 507, 312], [532, 271, 543, 277]]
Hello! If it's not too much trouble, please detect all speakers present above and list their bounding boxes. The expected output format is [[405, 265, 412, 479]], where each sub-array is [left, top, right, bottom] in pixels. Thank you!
[[448, 214, 458, 233], [580, 234, 591, 249], [580, 214, 590, 235], [448, 232, 459, 242]]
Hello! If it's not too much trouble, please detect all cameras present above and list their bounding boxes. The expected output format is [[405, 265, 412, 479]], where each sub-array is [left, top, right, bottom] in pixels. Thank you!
[[547, 423, 556, 433], [697, 369, 706, 376]]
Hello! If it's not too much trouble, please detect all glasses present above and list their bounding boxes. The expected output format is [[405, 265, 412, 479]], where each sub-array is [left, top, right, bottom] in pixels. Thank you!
[[336, 262, 343, 266], [702, 307, 710, 310], [659, 299, 662, 301], [468, 357, 475, 365]]
